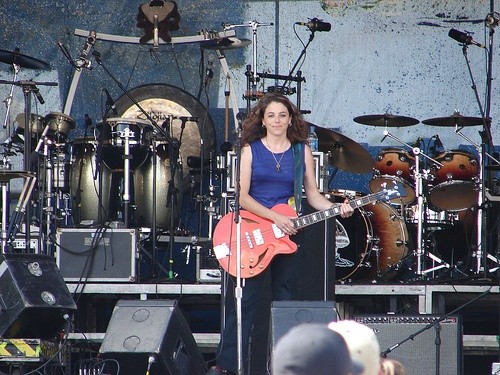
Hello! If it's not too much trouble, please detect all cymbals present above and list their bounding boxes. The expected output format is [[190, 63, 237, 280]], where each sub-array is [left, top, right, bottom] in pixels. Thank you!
[[0, 168, 34, 183], [422, 115, 492, 127], [354, 114, 420, 127], [0, 48, 56, 71], [201, 36, 251, 50], [304, 120, 375, 174]]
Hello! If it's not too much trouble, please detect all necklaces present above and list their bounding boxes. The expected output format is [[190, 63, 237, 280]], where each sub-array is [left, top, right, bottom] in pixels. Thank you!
[[265, 137, 288, 172]]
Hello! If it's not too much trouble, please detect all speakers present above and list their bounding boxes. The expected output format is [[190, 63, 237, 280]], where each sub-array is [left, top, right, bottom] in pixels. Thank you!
[[266, 195, 340, 375], [99, 298, 209, 375], [0, 253, 78, 341], [355, 313, 466, 375]]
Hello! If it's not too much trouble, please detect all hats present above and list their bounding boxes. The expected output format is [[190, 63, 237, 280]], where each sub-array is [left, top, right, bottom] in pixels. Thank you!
[[270, 319, 380, 375]]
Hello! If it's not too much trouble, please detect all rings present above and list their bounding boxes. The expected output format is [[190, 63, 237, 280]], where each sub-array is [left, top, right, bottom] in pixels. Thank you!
[[286, 229, 289, 231]]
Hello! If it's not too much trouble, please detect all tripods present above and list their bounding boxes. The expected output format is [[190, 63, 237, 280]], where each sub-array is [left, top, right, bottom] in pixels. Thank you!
[[371, 120, 500, 285]]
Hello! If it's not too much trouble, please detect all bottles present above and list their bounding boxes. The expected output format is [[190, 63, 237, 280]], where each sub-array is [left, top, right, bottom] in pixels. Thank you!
[[306, 126, 318, 152]]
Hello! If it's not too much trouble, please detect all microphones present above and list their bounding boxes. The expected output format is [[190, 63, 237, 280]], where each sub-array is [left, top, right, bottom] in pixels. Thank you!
[[33, 85, 45, 105], [58, 40, 76, 69], [104, 89, 118, 115], [448, 29, 485, 48], [435, 135, 443, 148], [380, 349, 391, 358], [297, 21, 332, 31], [85, 114, 92, 125], [205, 54, 215, 85], [35, 121, 51, 152], [173, 116, 200, 122]]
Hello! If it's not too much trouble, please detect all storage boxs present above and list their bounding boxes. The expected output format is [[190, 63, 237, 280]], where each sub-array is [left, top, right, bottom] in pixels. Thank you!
[[56, 228, 139, 282]]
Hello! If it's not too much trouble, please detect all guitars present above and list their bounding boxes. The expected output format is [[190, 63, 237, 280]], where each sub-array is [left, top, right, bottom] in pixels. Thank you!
[[213, 181, 409, 279]]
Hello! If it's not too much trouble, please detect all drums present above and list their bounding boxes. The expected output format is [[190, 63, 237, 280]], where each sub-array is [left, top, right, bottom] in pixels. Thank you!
[[15, 112, 43, 146], [36, 144, 74, 194], [99, 118, 153, 226], [400, 196, 456, 226], [327, 189, 408, 281], [69, 137, 112, 229], [370, 148, 424, 207], [427, 150, 480, 212], [46, 111, 75, 140], [134, 137, 184, 234]]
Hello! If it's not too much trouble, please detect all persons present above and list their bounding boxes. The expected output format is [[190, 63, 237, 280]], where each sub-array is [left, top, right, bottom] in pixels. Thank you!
[[380, 358, 406, 375], [216, 93, 354, 375], [330, 319, 379, 375], [272, 323, 363, 375]]
